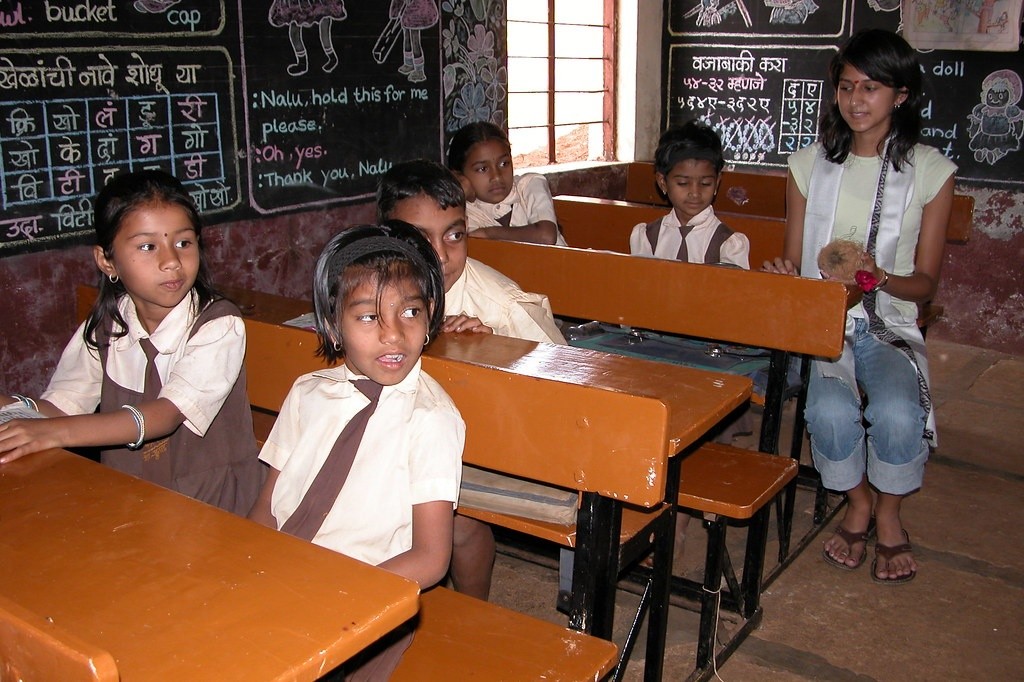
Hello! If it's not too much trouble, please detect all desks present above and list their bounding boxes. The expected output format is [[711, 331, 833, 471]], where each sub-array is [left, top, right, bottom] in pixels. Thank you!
[[196, 290, 754, 682], [0, 448, 421, 682]]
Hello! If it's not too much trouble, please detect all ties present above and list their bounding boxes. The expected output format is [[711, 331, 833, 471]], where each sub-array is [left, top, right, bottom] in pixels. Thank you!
[[676, 225, 694, 262], [494, 204, 514, 228], [138, 337, 162, 404], [277, 377, 385, 543]]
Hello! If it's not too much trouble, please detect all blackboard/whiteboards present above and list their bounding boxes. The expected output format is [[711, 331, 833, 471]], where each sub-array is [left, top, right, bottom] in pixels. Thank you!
[[0, 1, 509, 259], [660, 0, 1024, 192]]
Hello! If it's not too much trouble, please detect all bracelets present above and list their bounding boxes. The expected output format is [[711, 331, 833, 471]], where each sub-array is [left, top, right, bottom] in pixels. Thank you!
[[873, 270, 889, 290], [12, 393, 38, 412], [122, 404, 144, 449]]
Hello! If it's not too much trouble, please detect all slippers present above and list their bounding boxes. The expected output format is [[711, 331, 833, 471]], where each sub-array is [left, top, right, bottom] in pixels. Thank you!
[[822, 517, 878, 571], [870, 527, 916, 584]]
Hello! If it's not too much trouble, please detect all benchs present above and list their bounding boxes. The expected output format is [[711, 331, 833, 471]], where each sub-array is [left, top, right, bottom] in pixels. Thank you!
[[74, 285, 667, 682], [0, 594, 120, 682], [553, 195, 852, 525], [625, 163, 976, 345], [462, 237, 847, 670]]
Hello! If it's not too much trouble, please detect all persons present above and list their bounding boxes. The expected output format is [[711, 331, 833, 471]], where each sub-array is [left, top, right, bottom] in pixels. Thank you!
[[448, 121, 567, 246], [629, 121, 750, 566], [760, 28, 958, 584], [375, 157, 568, 601], [245, 219, 466, 682], [0, 170, 264, 519]]
